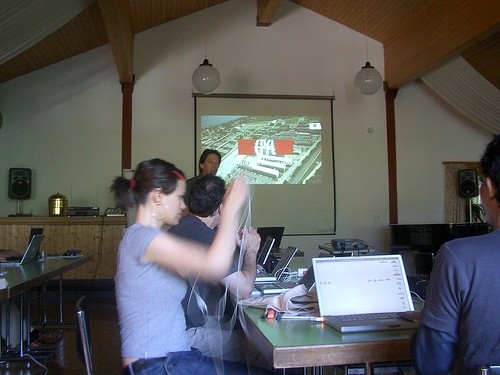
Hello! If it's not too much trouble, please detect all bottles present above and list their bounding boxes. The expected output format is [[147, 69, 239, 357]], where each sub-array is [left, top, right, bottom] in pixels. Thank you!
[[48, 192, 68, 216]]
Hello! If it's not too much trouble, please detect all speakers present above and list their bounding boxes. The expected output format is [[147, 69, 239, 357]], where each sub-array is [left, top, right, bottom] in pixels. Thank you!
[[8, 168, 32, 199], [458, 169, 479, 198]]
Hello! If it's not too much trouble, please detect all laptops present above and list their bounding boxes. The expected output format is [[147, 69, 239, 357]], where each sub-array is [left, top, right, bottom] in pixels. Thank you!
[[253, 255, 335, 294], [256, 236, 275, 265], [312, 254, 419, 332], [254, 246, 298, 281], [6, 228, 43, 261], [0, 234, 44, 265]]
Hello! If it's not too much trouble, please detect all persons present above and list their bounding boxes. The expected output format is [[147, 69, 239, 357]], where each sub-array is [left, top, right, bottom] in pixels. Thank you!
[[108, 159, 275, 375], [414, 132, 500, 375], [167, 174, 271, 369], [182, 149, 221, 214]]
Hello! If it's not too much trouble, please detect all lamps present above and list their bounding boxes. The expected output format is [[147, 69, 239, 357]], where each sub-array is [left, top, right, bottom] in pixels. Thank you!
[[355, 0, 382, 94], [190, 1, 221, 94]]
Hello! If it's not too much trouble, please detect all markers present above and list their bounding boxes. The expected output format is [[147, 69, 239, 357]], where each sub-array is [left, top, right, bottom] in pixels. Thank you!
[[276, 313, 283, 321]]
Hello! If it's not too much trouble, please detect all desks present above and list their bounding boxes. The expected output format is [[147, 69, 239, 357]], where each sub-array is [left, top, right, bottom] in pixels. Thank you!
[[0, 248, 93, 375], [0, 215, 127, 289], [228, 282, 423, 375], [256, 246, 302, 273]]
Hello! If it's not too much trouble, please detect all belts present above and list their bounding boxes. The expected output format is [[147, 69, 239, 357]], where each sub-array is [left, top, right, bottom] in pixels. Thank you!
[[122, 356, 164, 375]]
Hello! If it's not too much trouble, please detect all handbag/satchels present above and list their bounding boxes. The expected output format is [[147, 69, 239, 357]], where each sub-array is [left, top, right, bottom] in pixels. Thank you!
[[237, 284, 320, 315]]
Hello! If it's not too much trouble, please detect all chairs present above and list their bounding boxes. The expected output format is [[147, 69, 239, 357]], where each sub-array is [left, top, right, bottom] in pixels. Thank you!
[[73, 297, 99, 374]]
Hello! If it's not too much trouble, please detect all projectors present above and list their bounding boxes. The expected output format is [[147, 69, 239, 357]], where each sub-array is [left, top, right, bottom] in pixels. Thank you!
[[331, 239, 367, 250]]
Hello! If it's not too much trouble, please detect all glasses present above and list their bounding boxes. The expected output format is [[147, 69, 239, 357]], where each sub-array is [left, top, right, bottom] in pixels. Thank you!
[[478, 173, 497, 192]]
